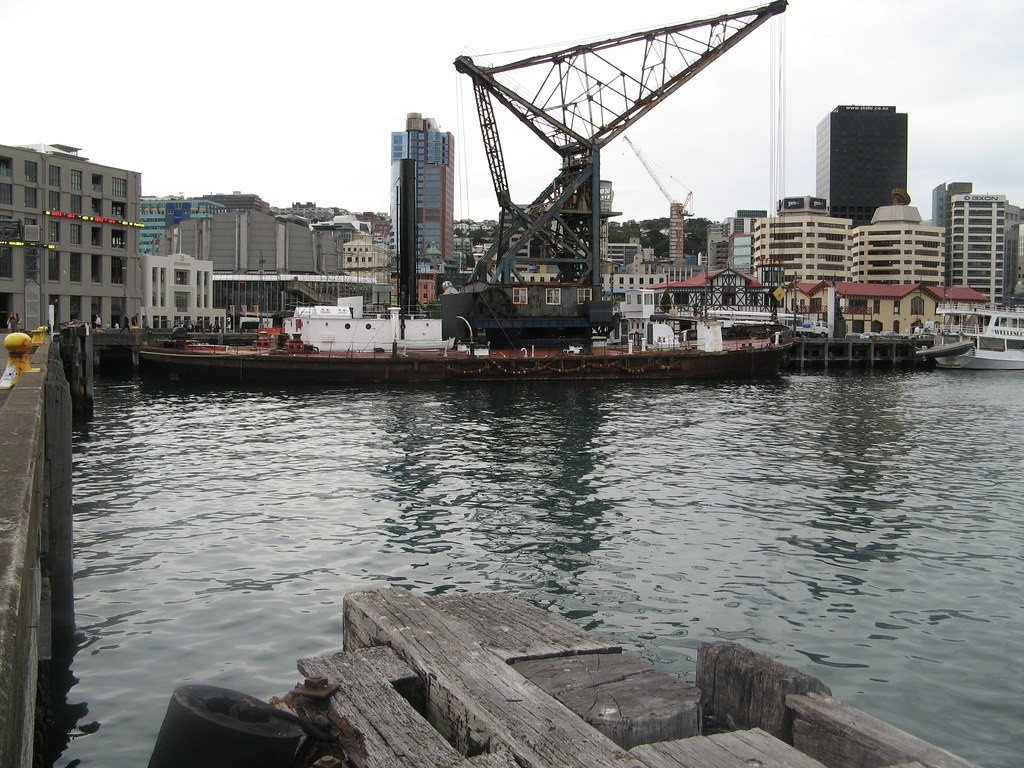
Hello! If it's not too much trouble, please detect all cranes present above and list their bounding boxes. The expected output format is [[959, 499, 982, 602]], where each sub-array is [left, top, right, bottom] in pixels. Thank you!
[[440, 0, 788, 349]]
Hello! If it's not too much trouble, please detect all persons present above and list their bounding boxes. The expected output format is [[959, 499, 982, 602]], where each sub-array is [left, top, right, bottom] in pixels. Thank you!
[[106, 322, 110, 329], [179, 318, 220, 333], [115, 323, 119, 329], [7, 312, 23, 334], [119, 313, 132, 333], [131, 313, 139, 326], [95, 313, 102, 330]]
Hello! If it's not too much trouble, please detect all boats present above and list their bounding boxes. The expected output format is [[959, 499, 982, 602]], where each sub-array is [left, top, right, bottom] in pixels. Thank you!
[[930, 302, 1024, 371], [280, 295, 455, 353]]
